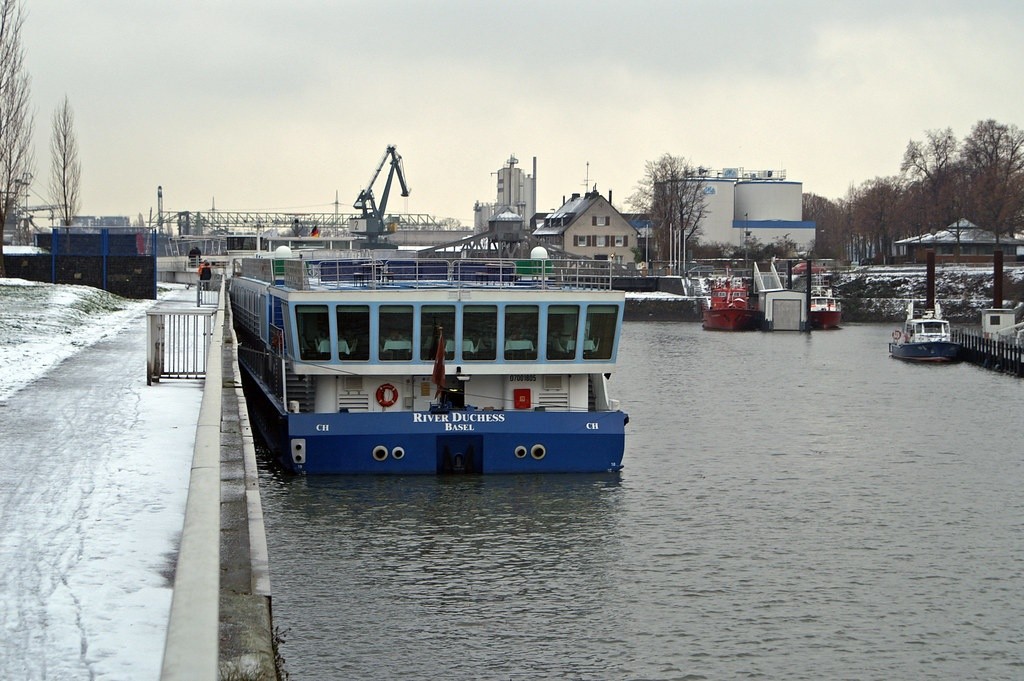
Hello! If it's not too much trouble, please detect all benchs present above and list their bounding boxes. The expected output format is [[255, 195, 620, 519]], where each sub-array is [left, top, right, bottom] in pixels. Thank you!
[[353, 272, 370, 287], [377, 273, 395, 287]]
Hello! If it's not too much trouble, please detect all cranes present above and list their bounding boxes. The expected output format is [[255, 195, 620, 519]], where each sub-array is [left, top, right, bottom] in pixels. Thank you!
[[348, 143, 412, 250]]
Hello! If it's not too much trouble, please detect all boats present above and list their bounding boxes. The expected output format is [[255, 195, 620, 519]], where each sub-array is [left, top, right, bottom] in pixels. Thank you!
[[699, 277, 760, 331], [807, 267, 843, 330], [887, 294, 962, 362], [231, 246, 631, 479]]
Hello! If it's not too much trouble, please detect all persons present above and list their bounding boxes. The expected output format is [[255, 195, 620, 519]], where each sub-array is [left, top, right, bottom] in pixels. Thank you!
[[725, 280, 730, 289], [904, 332, 913, 343], [188, 246, 201, 268], [199, 260, 212, 291]]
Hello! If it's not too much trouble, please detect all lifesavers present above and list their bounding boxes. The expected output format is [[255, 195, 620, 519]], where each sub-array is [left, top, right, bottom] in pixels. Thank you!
[[375, 384, 399, 407], [892, 330, 901, 340]]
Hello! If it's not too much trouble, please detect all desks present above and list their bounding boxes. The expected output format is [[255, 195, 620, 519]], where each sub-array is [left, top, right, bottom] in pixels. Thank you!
[[381, 335, 411, 360], [423, 334, 475, 360], [316, 336, 351, 359], [560, 338, 595, 359], [361, 263, 385, 288], [495, 337, 534, 360]]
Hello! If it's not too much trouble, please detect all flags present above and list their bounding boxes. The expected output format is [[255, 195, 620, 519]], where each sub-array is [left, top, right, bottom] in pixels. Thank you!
[[431, 332, 445, 399], [311, 226, 318, 237]]
